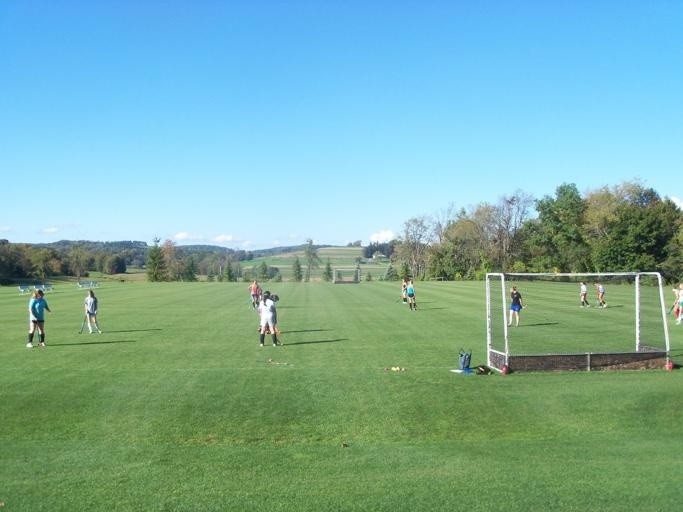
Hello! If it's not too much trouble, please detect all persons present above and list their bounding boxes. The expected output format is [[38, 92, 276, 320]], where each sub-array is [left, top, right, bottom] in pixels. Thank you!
[[21, 288, 51, 350], [248, 279, 259, 312], [401, 277, 406, 304], [591, 281, 608, 309], [578, 280, 590, 308], [256, 289, 277, 347], [506, 286, 525, 327], [256, 287, 281, 336], [81, 290, 102, 335], [406, 280, 416, 311], [671, 283, 682, 325]]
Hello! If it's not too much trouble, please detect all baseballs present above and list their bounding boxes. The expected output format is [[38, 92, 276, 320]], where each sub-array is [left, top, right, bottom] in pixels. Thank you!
[[391, 367, 400, 371], [384, 368, 388, 371], [402, 368, 405, 371]]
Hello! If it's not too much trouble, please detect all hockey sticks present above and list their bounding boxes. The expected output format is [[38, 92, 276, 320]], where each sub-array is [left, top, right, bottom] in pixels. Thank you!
[[78, 315, 87, 334], [277, 339, 284, 346], [666, 302, 675, 314]]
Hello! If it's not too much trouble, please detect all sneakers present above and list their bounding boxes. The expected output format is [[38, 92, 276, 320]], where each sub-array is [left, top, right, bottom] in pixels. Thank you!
[[273, 343, 276, 346], [39, 342, 46, 346], [580, 305, 584, 307], [599, 306, 603, 308], [90, 331, 92, 333], [587, 304, 590, 307], [260, 343, 263, 346], [98, 330, 101, 333], [27, 342, 34, 347], [604, 304, 607, 308]]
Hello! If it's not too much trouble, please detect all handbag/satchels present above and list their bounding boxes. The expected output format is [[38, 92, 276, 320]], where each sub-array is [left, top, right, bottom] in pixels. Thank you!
[[459, 349, 472, 369]]
